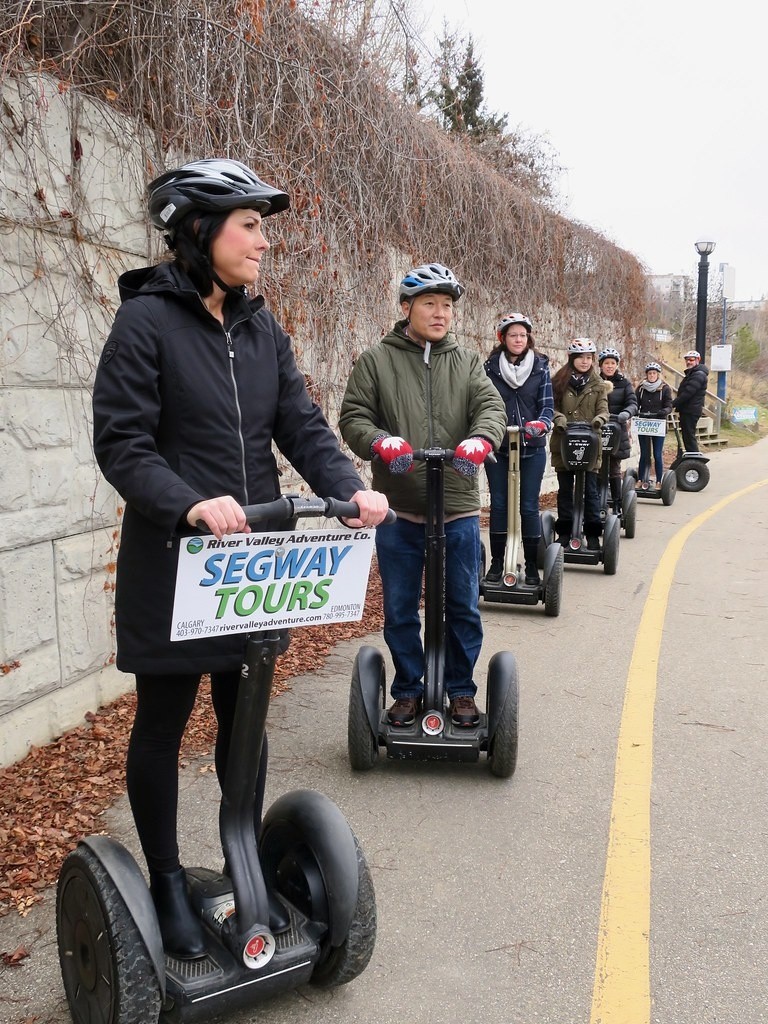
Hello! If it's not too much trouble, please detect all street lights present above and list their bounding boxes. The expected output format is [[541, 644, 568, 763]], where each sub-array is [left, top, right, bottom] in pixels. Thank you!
[[694, 241, 716, 417]]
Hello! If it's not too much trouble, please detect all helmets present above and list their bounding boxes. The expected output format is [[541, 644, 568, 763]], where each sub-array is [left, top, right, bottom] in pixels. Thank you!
[[146, 157, 291, 232], [496, 313, 532, 342], [683, 350, 701, 359], [598, 348, 621, 363], [568, 337, 597, 355], [645, 362, 661, 373], [399, 263, 465, 304]]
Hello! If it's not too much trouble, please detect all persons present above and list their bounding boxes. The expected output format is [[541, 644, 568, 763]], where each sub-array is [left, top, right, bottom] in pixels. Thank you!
[[549, 338, 613, 549], [484, 313, 554, 584], [598, 347, 640, 515], [92, 158, 389, 960], [337, 262, 508, 726], [671, 350, 709, 452], [634, 362, 673, 489]]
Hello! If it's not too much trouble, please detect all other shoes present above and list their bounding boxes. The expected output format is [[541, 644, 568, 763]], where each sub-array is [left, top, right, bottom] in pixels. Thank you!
[[635, 482, 642, 489], [655, 483, 662, 490], [586, 537, 600, 549], [555, 535, 570, 548]]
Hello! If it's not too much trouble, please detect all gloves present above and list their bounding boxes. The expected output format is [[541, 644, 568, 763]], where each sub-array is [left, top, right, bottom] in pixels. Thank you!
[[452, 436, 493, 476], [618, 411, 630, 423], [591, 417, 605, 431], [656, 409, 666, 418], [370, 432, 415, 475], [554, 416, 568, 431], [524, 420, 548, 439]]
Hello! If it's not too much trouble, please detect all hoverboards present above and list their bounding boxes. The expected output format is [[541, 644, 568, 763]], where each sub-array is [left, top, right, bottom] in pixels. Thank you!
[[622, 411, 676, 506], [479, 425, 564, 617], [348, 446, 518, 778], [670, 400, 710, 493], [540, 421, 620, 575], [56, 496, 397, 1023], [603, 414, 637, 538]]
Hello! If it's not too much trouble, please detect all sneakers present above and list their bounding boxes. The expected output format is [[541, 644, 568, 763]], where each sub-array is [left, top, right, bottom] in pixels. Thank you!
[[387, 696, 421, 727], [449, 696, 480, 727], [486, 563, 504, 581], [525, 560, 540, 585]]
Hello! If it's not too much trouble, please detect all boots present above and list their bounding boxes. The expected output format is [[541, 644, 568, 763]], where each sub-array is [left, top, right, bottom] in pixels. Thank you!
[[612, 501, 622, 518]]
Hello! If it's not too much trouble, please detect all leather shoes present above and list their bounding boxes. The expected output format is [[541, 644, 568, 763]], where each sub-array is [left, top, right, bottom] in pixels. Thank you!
[[151, 869, 210, 962], [267, 890, 292, 934]]
